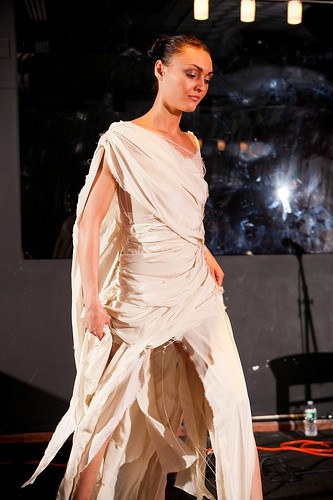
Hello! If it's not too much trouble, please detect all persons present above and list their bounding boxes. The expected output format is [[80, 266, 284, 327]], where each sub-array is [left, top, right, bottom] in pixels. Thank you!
[[56, 32, 267, 500]]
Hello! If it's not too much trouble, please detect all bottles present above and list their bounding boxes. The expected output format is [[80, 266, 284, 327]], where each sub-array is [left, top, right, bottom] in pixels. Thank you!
[[304, 400, 318, 437]]
